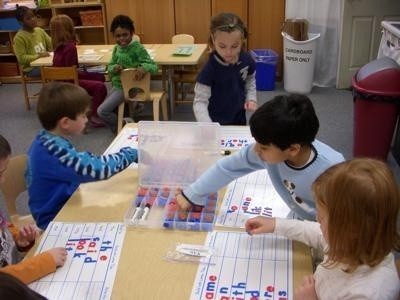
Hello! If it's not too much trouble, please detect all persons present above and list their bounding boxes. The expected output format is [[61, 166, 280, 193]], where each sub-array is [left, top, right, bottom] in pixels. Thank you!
[[0, 135, 68, 285], [25, 82, 154, 230], [192, 12, 260, 125], [245, 157, 400, 300], [97, 15, 158, 135], [49, 14, 106, 128], [175, 93, 345, 221], [12, 4, 54, 78]]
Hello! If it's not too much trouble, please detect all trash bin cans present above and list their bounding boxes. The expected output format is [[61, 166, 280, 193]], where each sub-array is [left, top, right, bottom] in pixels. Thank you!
[[248, 49, 278, 91], [352, 57, 400, 162]]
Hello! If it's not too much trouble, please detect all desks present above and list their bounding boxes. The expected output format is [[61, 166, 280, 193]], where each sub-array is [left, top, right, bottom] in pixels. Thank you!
[[30, 42, 212, 124], [21, 120, 316, 300]]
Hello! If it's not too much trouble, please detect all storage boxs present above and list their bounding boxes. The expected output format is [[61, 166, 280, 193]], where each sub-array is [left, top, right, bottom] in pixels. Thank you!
[[0, 17, 21, 32], [77, 9, 104, 28]]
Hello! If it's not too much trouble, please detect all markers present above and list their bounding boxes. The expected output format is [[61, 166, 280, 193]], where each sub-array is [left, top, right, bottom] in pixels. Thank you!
[[138, 203, 150, 220], [130, 203, 142, 224]]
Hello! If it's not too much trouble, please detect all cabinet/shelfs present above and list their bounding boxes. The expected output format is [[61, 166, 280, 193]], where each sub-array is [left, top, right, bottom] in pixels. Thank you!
[[247, 0, 285, 82], [173, 1, 249, 53], [0, 0, 109, 85], [104, 1, 176, 46]]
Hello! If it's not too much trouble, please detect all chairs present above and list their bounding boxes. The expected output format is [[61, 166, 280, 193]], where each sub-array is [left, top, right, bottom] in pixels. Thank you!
[[132, 34, 141, 45], [39, 63, 95, 135], [9, 32, 44, 111], [166, 32, 198, 107], [114, 68, 170, 137], [0, 153, 46, 260]]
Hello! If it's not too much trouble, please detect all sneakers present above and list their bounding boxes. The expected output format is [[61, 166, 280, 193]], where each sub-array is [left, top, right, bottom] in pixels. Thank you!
[[89, 117, 105, 126], [122, 116, 134, 123]]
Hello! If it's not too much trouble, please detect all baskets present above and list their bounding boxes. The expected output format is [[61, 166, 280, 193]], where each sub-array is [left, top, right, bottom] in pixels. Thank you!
[[0, 41, 11, 54], [79, 10, 102, 25], [36, 15, 50, 27]]
